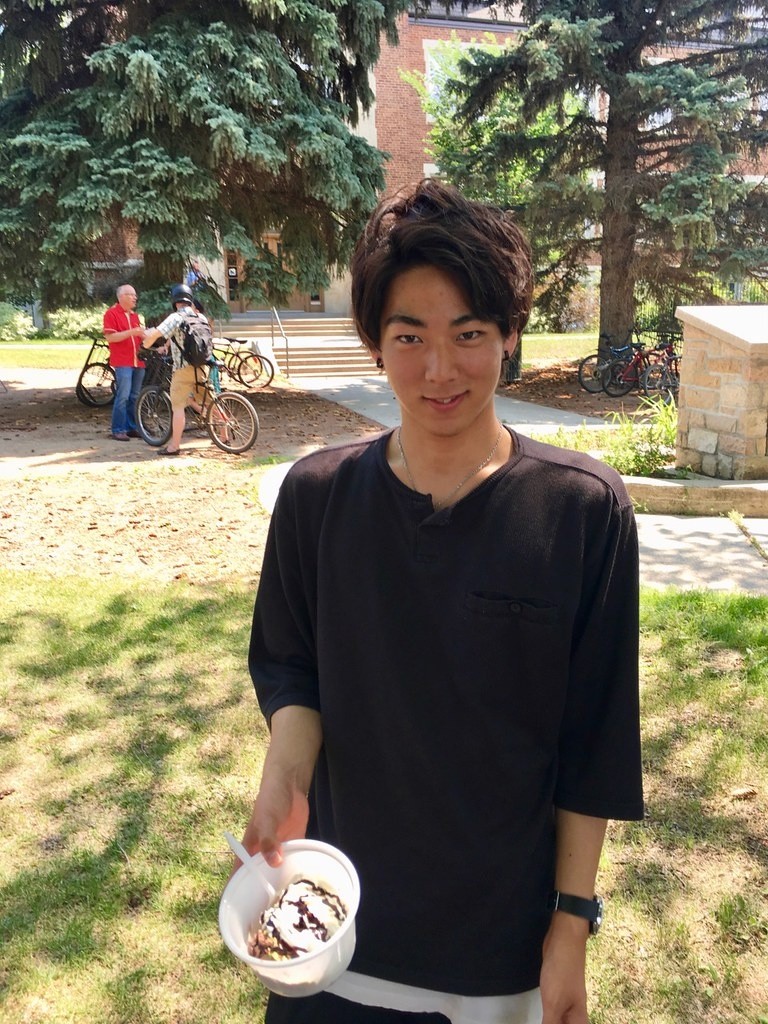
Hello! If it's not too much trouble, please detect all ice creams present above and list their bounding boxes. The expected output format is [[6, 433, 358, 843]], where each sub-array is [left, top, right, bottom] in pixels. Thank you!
[[249, 876, 347, 960]]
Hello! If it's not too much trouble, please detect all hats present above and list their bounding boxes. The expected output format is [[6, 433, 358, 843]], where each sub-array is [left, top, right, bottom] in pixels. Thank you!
[[193, 296, 205, 311]]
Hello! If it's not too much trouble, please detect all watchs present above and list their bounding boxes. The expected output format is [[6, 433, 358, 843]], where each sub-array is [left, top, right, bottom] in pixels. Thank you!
[[551, 889, 605, 935]]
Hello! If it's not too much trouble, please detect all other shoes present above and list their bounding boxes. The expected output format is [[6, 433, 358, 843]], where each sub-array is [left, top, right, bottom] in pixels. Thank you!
[[157, 446, 180, 455], [127, 430, 150, 437], [196, 430, 209, 438], [113, 432, 130, 441], [211, 440, 231, 446]]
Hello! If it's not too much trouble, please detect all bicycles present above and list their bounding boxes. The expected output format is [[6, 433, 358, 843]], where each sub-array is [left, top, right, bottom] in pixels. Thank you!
[[209, 336, 275, 389], [577, 324, 682, 408], [133, 348, 260, 455], [75, 334, 172, 408]]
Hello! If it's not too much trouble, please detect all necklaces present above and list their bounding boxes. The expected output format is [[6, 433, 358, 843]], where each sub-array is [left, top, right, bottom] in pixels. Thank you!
[[396, 419, 503, 507]]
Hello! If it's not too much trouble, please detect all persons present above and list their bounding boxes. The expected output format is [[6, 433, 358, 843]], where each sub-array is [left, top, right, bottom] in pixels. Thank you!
[[232, 178, 645, 1024], [187, 262, 207, 309], [143, 284, 231, 455], [103, 284, 147, 439]]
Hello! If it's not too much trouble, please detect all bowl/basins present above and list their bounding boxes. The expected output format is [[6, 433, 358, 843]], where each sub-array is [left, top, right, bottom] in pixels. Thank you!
[[219, 838, 361, 997]]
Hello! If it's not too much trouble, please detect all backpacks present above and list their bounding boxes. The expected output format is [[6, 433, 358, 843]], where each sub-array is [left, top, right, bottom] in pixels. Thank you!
[[171, 311, 214, 367]]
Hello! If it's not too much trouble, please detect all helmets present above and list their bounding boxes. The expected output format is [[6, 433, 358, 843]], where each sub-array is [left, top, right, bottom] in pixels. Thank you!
[[171, 284, 194, 312]]
